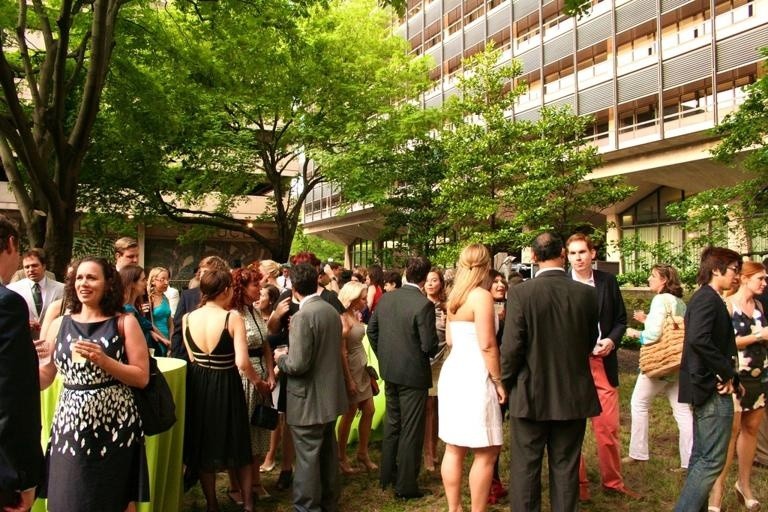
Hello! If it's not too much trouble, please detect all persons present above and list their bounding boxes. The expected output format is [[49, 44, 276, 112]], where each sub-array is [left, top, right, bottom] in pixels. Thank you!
[[253, 259, 290, 321], [499, 232, 603, 511], [383, 271, 400, 293], [111, 237, 140, 273], [170, 256, 232, 360], [39, 259, 81, 343], [505, 271, 526, 294], [318, 262, 366, 314], [6, 248, 66, 340], [621, 264, 693, 473], [146, 268, 174, 355], [267, 253, 343, 492], [482, 269, 508, 346], [255, 284, 279, 320], [183, 270, 257, 512], [0, 216, 43, 512], [319, 261, 365, 293], [708, 262, 768, 511], [226, 267, 274, 512], [565, 235, 644, 506], [338, 282, 380, 476], [366, 265, 384, 313], [119, 266, 165, 357], [274, 264, 350, 511], [674, 248, 745, 512], [424, 266, 447, 484], [438, 244, 506, 512], [366, 256, 439, 501], [33, 257, 150, 512]]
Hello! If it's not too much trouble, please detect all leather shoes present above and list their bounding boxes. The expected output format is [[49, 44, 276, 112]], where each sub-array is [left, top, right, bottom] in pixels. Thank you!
[[394, 489, 432, 499], [603, 486, 645, 502], [579, 483, 592, 505]]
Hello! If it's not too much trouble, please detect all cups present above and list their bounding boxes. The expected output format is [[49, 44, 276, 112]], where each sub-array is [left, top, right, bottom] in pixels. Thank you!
[[277, 344, 287, 353], [71, 338, 91, 364], [633, 307, 644, 312]]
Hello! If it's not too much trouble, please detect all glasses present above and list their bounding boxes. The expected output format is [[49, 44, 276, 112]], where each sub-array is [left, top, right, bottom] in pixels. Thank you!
[[729, 266, 740, 276], [154, 277, 171, 284]]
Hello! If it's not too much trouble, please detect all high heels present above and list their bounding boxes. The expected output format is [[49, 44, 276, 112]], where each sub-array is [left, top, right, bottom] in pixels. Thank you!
[[708, 506, 719, 511], [734, 481, 762, 511], [275, 471, 294, 491], [425, 466, 436, 474], [622, 456, 638, 465], [259, 461, 277, 473], [338, 461, 356, 472], [252, 486, 272, 503], [226, 487, 243, 508], [432, 458, 439, 463], [489, 484, 509, 505], [670, 468, 690, 475], [356, 458, 377, 470]]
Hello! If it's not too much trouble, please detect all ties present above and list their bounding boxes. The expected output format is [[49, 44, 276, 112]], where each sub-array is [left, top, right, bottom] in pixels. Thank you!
[[34, 283, 44, 317]]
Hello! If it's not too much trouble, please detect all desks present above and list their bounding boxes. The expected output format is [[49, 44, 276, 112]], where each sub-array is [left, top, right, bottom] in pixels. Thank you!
[[34, 356, 189, 511]]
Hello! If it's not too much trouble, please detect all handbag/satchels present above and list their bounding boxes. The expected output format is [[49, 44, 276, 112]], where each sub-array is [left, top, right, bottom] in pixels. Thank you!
[[250, 392, 280, 430], [638, 295, 685, 379], [367, 366, 379, 396], [120, 312, 176, 437]]
